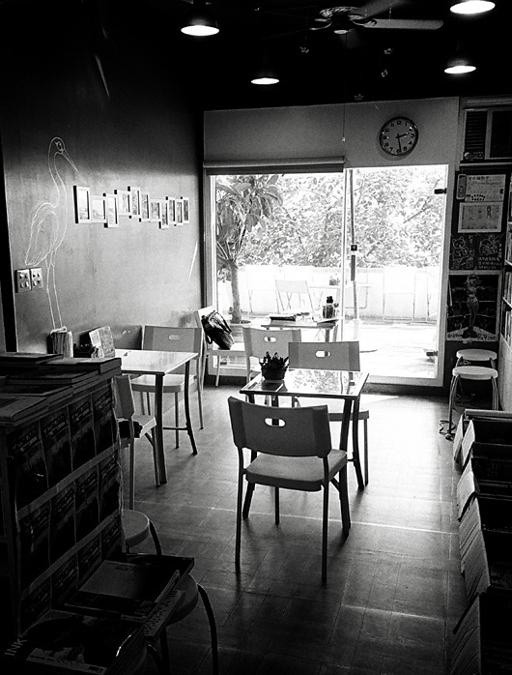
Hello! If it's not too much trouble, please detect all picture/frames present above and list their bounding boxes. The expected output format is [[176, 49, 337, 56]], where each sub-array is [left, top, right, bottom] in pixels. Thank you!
[[73, 185, 189, 229]]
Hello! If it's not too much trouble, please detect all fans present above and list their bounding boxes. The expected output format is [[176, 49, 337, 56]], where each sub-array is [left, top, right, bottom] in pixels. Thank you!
[[308, 0, 443, 34]]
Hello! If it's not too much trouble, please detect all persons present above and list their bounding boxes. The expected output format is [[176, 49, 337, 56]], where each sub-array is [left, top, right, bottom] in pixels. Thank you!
[[464, 275, 483, 338]]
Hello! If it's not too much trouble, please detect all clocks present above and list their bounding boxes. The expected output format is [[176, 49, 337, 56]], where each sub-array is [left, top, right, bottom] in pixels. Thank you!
[[379, 116, 418, 156]]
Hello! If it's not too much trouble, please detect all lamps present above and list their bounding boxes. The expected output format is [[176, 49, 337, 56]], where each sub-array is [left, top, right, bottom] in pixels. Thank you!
[[450, 0, 496, 15], [179, 0, 220, 37], [444, 59, 478, 75], [250, 71, 280, 85]]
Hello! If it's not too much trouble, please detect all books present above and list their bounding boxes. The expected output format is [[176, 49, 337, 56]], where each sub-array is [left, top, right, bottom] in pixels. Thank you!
[[0, 349, 197, 674], [496, 179, 511, 347], [452, 405, 510, 673]]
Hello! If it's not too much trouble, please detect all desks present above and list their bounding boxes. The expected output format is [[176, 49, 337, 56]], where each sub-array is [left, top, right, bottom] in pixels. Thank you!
[[0, 376, 125, 675]]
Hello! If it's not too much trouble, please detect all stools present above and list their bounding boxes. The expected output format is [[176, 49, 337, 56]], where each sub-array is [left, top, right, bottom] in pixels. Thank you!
[[444, 349, 499, 440], [118, 508, 219, 675]]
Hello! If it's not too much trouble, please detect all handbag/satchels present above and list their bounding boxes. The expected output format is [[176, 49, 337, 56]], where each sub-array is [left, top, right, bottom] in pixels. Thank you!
[[202, 311, 235, 350]]
